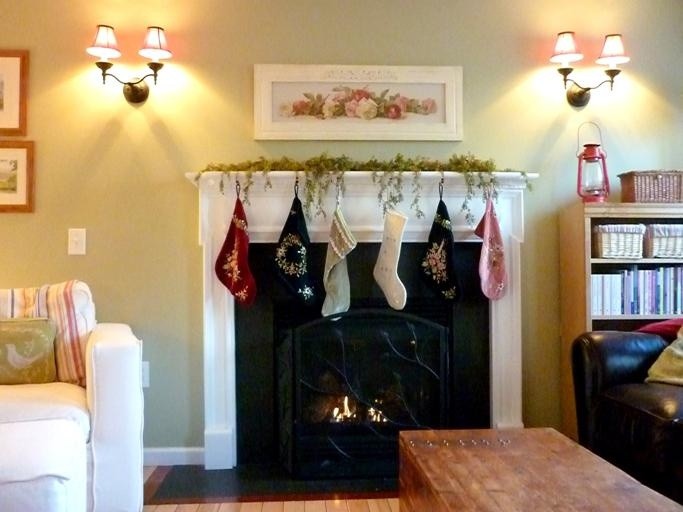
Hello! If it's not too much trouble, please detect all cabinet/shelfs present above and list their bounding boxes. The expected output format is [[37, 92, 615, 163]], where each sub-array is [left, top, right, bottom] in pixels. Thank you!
[[554, 199, 683, 440]]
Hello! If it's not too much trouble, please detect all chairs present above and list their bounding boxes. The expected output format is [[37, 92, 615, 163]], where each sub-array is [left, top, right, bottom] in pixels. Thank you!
[[569, 316, 683, 503], [0, 278, 147, 512]]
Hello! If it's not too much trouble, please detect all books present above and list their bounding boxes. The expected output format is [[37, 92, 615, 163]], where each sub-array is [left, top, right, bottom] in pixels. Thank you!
[[590, 265, 683, 315]]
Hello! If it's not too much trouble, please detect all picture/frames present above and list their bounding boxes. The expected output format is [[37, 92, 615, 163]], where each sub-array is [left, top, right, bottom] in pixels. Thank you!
[[0, 138, 35, 217], [250, 61, 464, 142], [0, 48, 29, 138]]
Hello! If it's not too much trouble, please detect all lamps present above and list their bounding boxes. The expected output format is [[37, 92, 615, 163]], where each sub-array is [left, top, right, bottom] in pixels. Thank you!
[[573, 122, 611, 205], [546, 29, 631, 109], [85, 24, 173, 106]]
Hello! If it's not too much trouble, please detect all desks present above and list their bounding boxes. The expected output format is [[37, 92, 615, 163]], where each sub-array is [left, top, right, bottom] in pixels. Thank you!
[[394, 426, 683, 512]]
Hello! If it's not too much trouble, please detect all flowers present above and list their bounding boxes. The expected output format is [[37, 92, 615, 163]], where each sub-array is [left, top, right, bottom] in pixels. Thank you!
[[276, 89, 439, 121]]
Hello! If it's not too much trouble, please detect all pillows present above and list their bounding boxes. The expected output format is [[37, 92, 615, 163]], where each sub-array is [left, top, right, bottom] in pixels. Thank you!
[[645, 325, 683, 386], [0, 314, 60, 382]]
